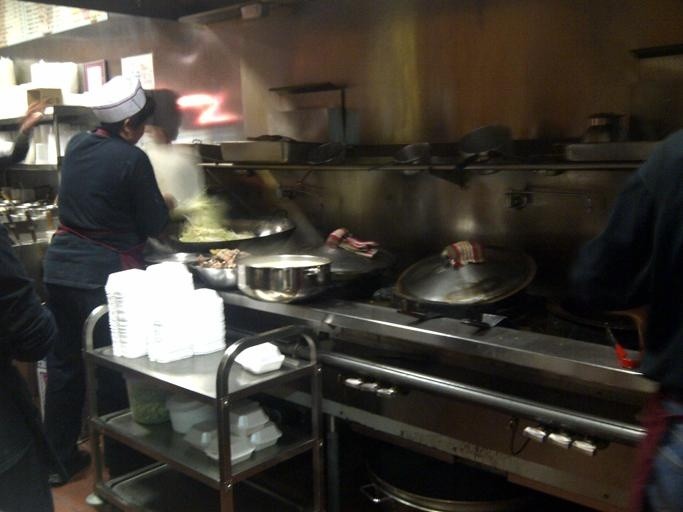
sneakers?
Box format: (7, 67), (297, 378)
(48, 450), (91, 487)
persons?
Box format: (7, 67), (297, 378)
(129, 87), (208, 212)
(0, 222), (69, 511)
(587, 128), (682, 511)
(34, 73), (192, 490)
(0, 99), (45, 173)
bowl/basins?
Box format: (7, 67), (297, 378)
(0, 198), (61, 220)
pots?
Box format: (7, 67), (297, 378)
(393, 239), (539, 325)
(448, 122), (512, 172)
(184, 256), (238, 288)
(145, 251), (209, 271)
(366, 140), (433, 172)
(295, 140), (346, 186)
(145, 211), (298, 255)
(308, 240), (398, 304)
(236, 255), (338, 303)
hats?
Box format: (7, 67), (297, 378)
(90, 75), (147, 124)
(628, 42), (683, 74)
